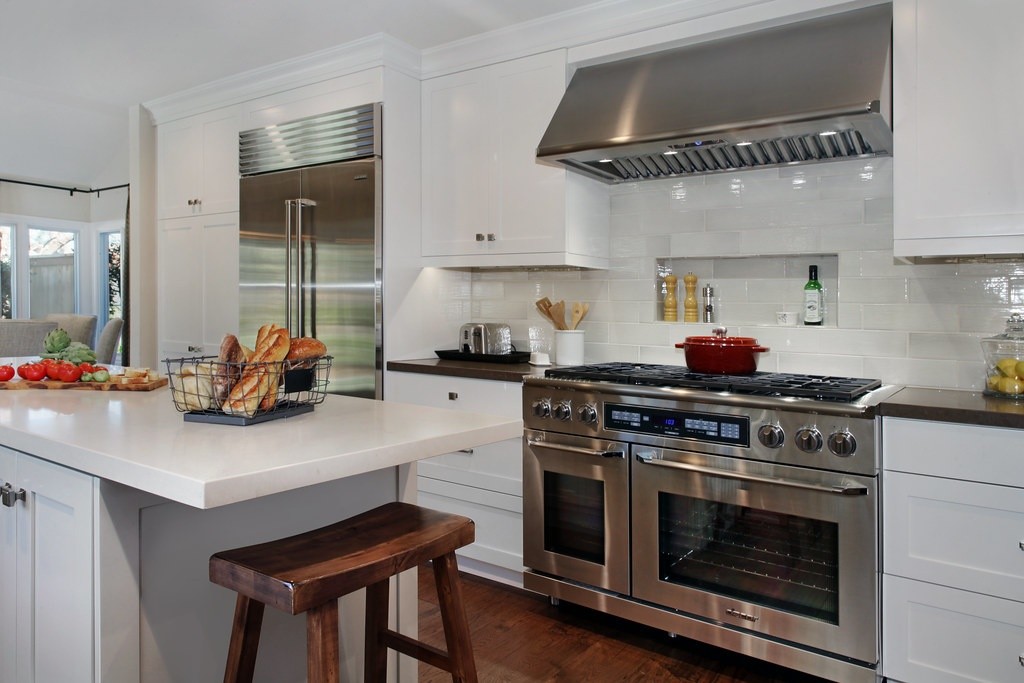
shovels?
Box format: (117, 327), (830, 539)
(536, 297), (559, 329)
(571, 303), (588, 329)
(549, 300), (568, 329)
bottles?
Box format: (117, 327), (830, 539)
(702, 283), (715, 323)
(980, 312), (1024, 401)
(803, 264), (824, 325)
(684, 271), (698, 323)
(664, 272), (677, 322)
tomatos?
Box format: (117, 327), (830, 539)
(0, 357), (112, 382)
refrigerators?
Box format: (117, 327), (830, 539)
(238, 103), (383, 400)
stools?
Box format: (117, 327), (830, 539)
(209, 502), (479, 683)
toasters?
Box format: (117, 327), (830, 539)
(458, 321), (511, 355)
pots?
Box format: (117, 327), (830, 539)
(675, 326), (770, 376)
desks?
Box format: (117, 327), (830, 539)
(0, 357), (523, 683)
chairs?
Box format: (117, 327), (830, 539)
(0, 314), (123, 365)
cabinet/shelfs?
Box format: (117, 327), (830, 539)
(880, 388), (1024, 683)
(158, 114), (239, 375)
(893, 0), (1024, 256)
(0, 447), (93, 683)
(420, 48), (610, 270)
(386, 354), (573, 597)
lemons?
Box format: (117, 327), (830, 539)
(987, 356), (1024, 394)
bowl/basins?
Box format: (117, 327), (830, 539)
(776, 312), (799, 325)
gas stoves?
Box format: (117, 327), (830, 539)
(520, 360), (907, 476)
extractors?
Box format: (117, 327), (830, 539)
(535, 3), (894, 184)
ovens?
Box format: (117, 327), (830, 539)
(521, 428), (884, 682)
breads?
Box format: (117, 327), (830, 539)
(110, 367), (159, 385)
(172, 322), (326, 418)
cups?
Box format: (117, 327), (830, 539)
(554, 329), (586, 366)
